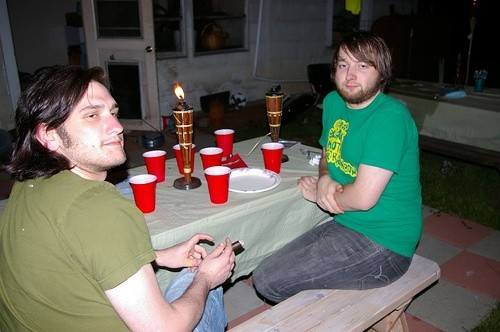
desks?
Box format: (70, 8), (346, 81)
(384, 79), (500, 153)
(0, 135), (335, 294)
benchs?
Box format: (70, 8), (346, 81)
(224, 252), (441, 332)
(418, 134), (500, 170)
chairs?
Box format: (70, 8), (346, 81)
(301, 63), (336, 125)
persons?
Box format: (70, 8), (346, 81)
(252, 31), (423, 303)
(0, 65), (235, 332)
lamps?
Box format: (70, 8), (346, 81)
(266, 85), (289, 163)
(172, 84), (203, 191)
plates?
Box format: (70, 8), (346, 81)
(228, 167), (282, 194)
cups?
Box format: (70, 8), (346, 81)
(199, 147), (224, 176)
(172, 144), (196, 175)
(214, 129), (234, 158)
(203, 166), (231, 204)
(261, 143), (284, 174)
(143, 150), (166, 183)
(129, 174), (158, 214)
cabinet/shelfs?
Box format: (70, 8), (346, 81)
(153, 0), (250, 60)
